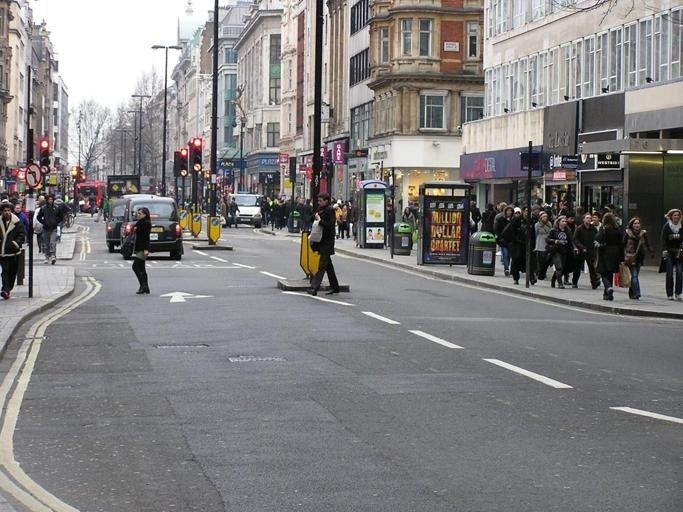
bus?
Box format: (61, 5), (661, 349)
(75, 180), (105, 213)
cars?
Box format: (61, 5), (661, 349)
(120, 194), (157, 199)
(103, 198), (129, 252)
(116, 196), (184, 261)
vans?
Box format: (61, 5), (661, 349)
(225, 194), (262, 228)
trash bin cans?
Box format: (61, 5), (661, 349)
(288, 211), (301, 233)
(390, 222), (411, 255)
(468, 231), (496, 276)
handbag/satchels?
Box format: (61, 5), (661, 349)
(620, 263), (633, 289)
(120, 233), (134, 259)
(624, 253), (637, 266)
(546, 243), (559, 251)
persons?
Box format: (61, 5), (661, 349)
(0, 191), (110, 300)
(658, 209), (683, 300)
(261, 195), (358, 240)
(367, 229), (375, 240)
(306, 192), (340, 296)
(469, 198), (655, 300)
(374, 229), (383, 240)
(387, 199), (420, 247)
(184, 197), (241, 228)
(131, 208), (151, 294)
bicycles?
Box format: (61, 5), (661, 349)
(61, 213), (74, 228)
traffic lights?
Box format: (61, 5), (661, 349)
(77, 167), (83, 181)
(190, 137), (202, 172)
(180, 149), (188, 177)
(39, 139), (50, 166)
(324, 161), (333, 180)
(72, 166), (77, 179)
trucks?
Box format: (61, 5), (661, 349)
(139, 177), (155, 194)
(102, 175), (140, 205)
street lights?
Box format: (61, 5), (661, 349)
(107, 110), (144, 176)
(131, 95), (150, 177)
(151, 46), (182, 197)
(231, 114), (242, 193)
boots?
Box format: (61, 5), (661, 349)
(136, 272), (150, 295)
